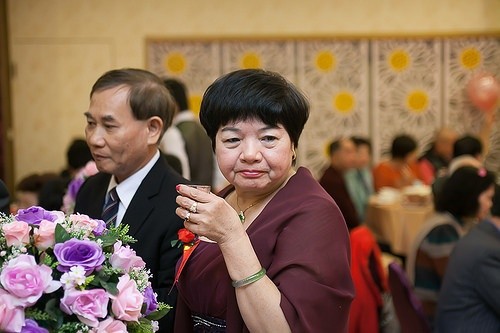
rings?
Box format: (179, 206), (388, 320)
(184, 212), (191, 221)
(188, 201), (198, 213)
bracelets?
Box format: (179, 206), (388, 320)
(231, 267), (266, 288)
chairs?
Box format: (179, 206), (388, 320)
(386, 262), (433, 333)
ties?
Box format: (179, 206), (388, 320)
(101, 186), (121, 230)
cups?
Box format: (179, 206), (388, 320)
(182, 185), (212, 211)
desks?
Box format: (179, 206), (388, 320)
(365, 191), (434, 258)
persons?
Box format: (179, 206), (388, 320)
(158, 68), (356, 333)
(319, 132), (500, 333)
(0, 68), (233, 333)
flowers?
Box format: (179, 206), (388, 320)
(0, 204), (173, 333)
(171, 228), (200, 251)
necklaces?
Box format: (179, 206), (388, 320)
(235, 190), (275, 225)
